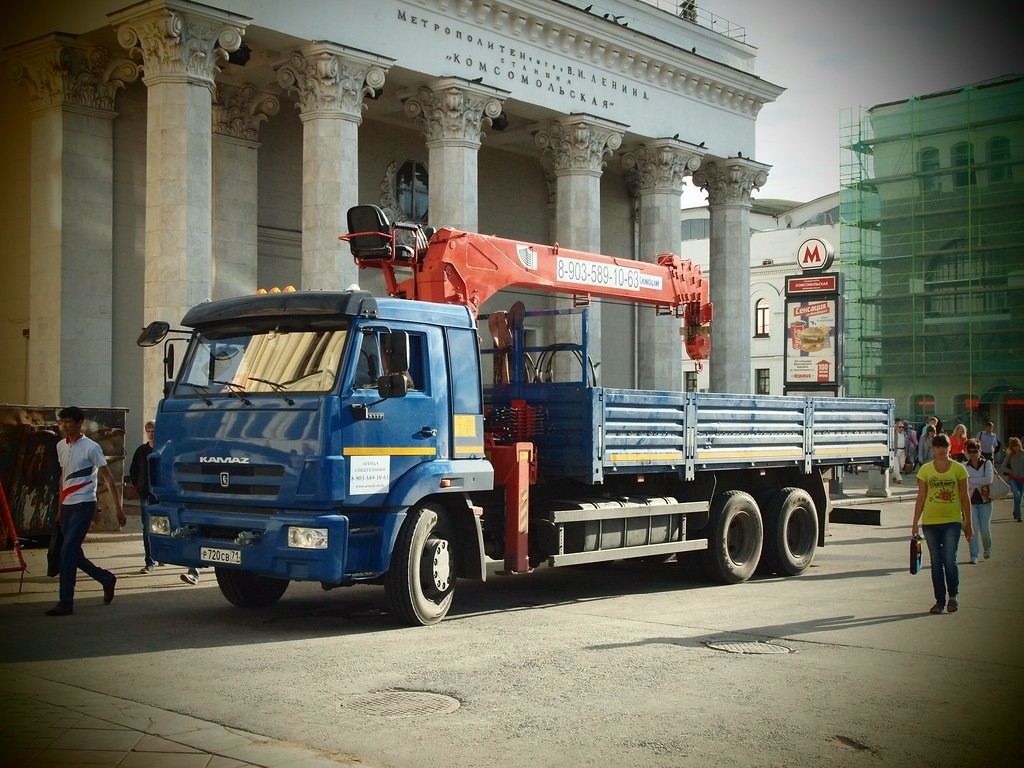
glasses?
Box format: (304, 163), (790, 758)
(968, 449), (980, 454)
(899, 426), (904, 428)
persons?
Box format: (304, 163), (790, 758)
(912, 433), (973, 613)
(46, 405), (126, 616)
(180, 568), (199, 586)
(369, 340), (415, 390)
(891, 416), (1024, 523)
(962, 437), (994, 564)
(128, 419), (165, 575)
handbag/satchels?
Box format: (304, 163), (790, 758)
(984, 459), (1011, 501)
(47, 522), (64, 577)
(910, 536), (922, 574)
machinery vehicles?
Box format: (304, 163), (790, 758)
(138, 204), (894, 624)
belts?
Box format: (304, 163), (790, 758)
(897, 447), (905, 449)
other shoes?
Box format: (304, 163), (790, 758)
(969, 558), (977, 564)
(140, 565), (155, 574)
(984, 547), (992, 559)
(103, 574), (116, 605)
(1017, 517), (1022, 522)
(45, 606), (73, 616)
(180, 573), (198, 585)
(893, 477), (903, 484)
(1014, 512), (1017, 519)
(947, 595), (959, 612)
(930, 605), (944, 614)
